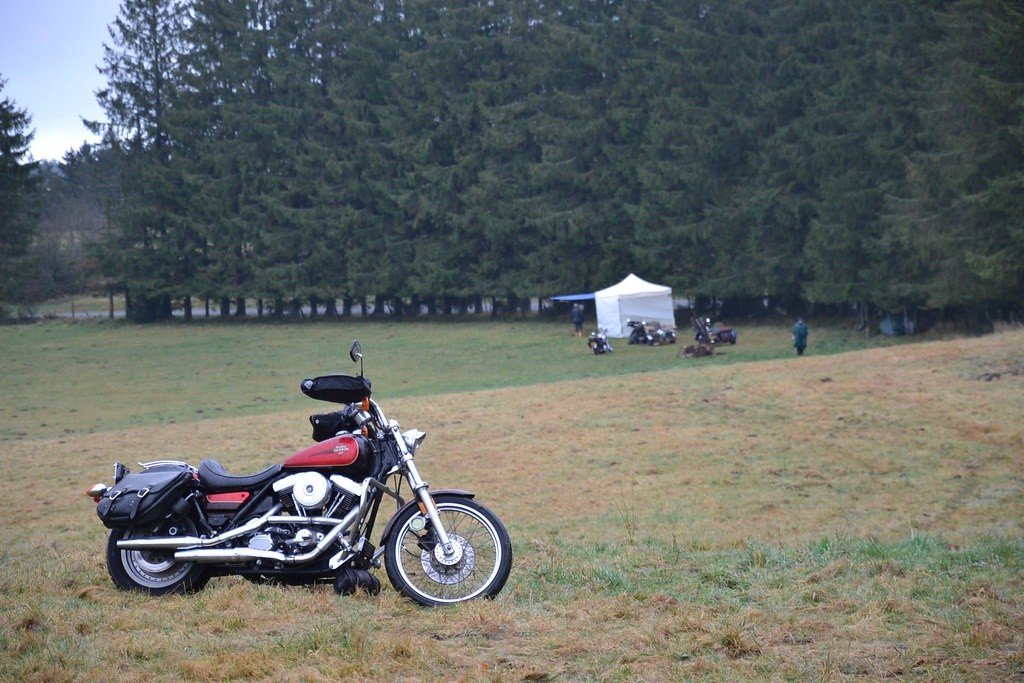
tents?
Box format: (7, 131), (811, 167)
(549, 272), (676, 338)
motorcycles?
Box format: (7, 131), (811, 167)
(87, 339), (515, 606)
(625, 319), (676, 346)
(713, 322), (737, 345)
(587, 327), (613, 356)
(692, 317), (720, 345)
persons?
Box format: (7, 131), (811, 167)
(792, 317), (809, 356)
(571, 303), (585, 338)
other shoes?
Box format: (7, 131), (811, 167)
(579, 333), (581, 336)
(575, 332), (578, 336)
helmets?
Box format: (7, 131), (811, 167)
(574, 303), (578, 308)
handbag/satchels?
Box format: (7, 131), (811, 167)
(96, 471), (193, 527)
